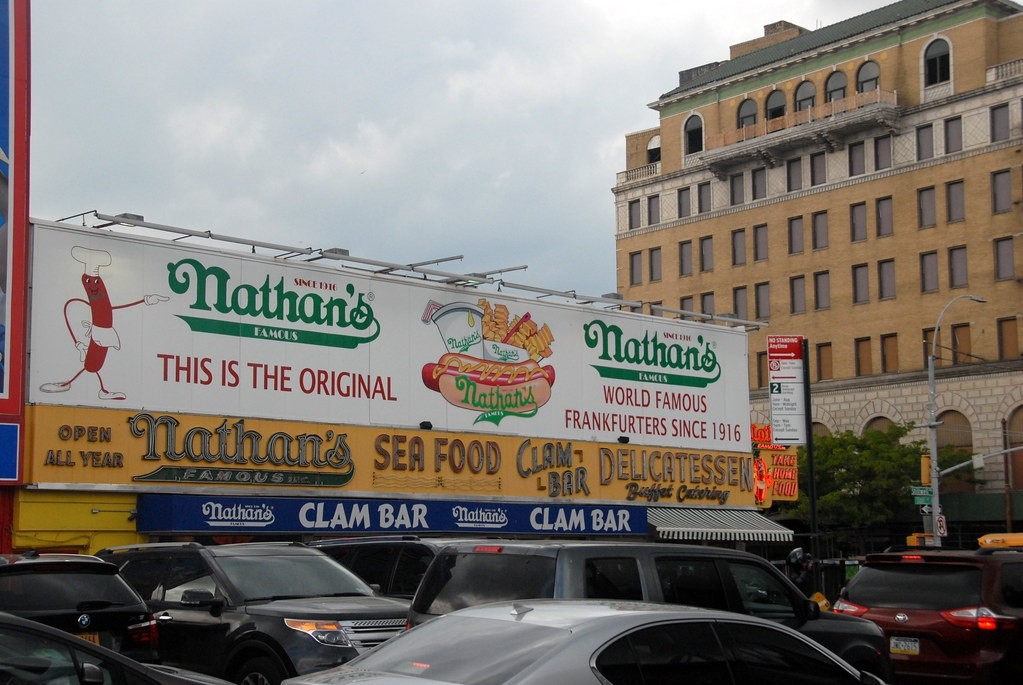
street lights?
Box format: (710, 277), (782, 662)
(924, 294), (989, 548)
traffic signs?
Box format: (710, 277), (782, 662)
(765, 334), (809, 447)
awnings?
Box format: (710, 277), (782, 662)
(646, 508), (796, 545)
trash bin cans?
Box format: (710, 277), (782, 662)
(820, 558), (860, 602)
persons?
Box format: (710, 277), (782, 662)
(788, 544), (811, 583)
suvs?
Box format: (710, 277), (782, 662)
(831, 546), (1022, 685)
(287, 543), (891, 685)
(2, 537), (497, 685)
